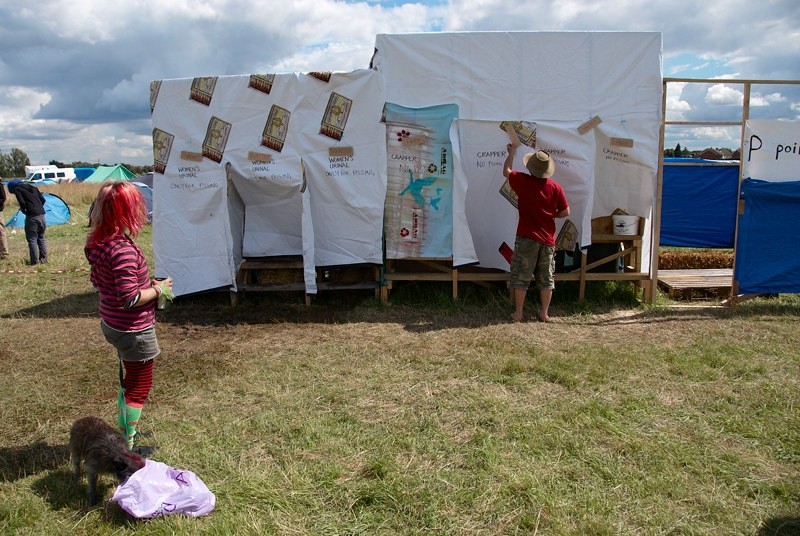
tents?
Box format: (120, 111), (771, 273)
(35, 179), (55, 184)
(4, 193), (71, 227)
(82, 164), (138, 183)
(132, 172), (153, 189)
(132, 182), (153, 224)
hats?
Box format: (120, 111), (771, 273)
(7, 178), (22, 194)
(523, 149), (555, 178)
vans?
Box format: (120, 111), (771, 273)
(21, 167), (77, 183)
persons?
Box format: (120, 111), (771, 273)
(0, 180), (9, 258)
(503, 142), (570, 322)
(7, 179), (46, 266)
(83, 181), (173, 458)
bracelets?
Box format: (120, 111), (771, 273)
(153, 285), (162, 297)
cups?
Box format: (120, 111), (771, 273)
(151, 278), (167, 311)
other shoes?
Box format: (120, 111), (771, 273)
(27, 262), (36, 265)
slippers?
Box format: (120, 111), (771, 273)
(507, 313), (520, 322)
(537, 309), (547, 323)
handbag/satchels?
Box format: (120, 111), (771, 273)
(110, 458), (216, 518)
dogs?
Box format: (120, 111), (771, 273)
(69, 417), (144, 506)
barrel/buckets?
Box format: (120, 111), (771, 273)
(612, 214), (641, 236)
